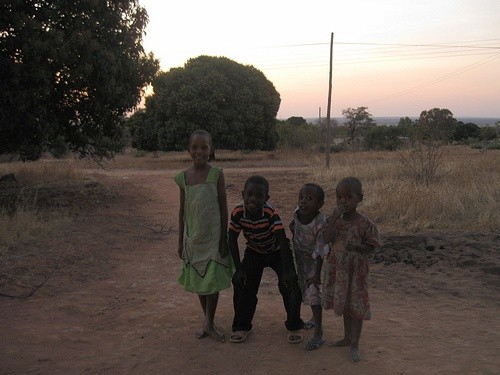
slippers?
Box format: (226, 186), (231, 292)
(287, 332), (303, 344)
(304, 320), (316, 330)
(230, 329), (251, 343)
(303, 339), (327, 350)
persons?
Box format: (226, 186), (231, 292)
(322, 176), (382, 361)
(289, 183), (331, 351)
(228, 176), (303, 344)
(172, 130), (226, 344)
